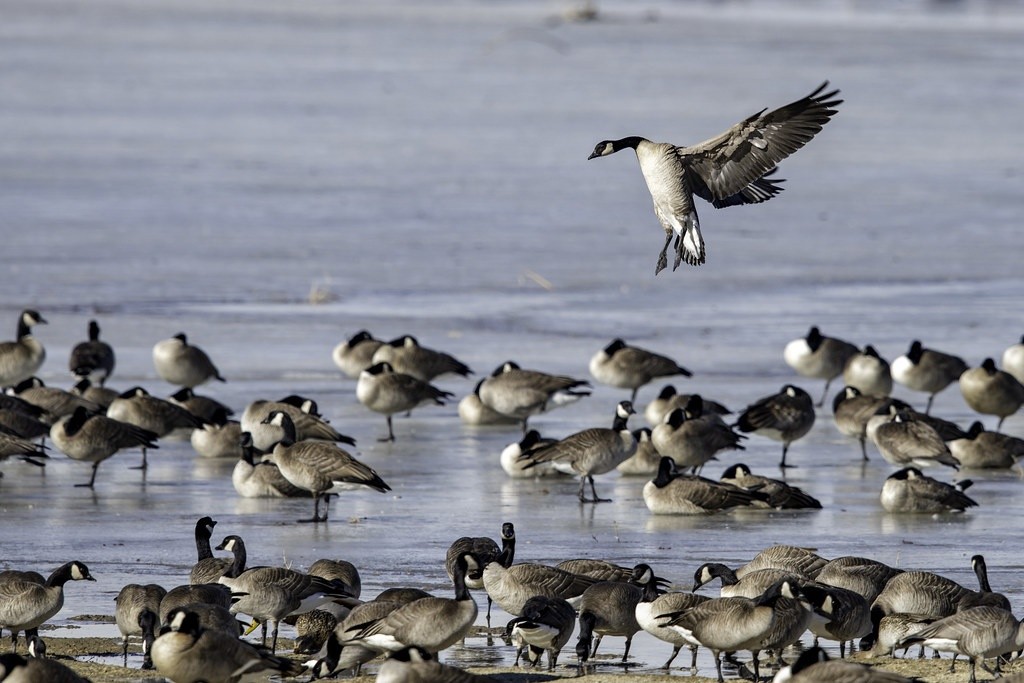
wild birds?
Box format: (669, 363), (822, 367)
(459, 361), (596, 440)
(616, 325), (1024, 516)
(231, 431), (339, 498)
(0, 515), (1024, 683)
(106, 387), (205, 470)
(590, 338), (694, 404)
(587, 79), (844, 276)
(239, 394), (357, 454)
(513, 401), (637, 504)
(169, 387), (236, 426)
(68, 321), (115, 386)
(152, 333), (226, 386)
(189, 417), (242, 457)
(332, 329), (384, 379)
(0, 309), (106, 479)
(372, 334), (476, 419)
(499, 430), (570, 479)
(47, 405), (160, 487)
(260, 411), (392, 523)
(356, 363), (455, 442)
